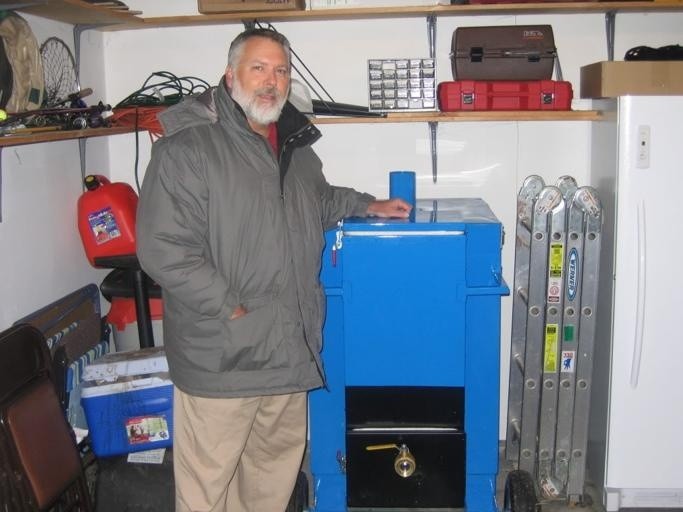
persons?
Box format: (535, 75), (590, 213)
(135, 30), (413, 512)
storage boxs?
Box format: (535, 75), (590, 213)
(78, 345), (172, 458)
(580, 61), (683, 98)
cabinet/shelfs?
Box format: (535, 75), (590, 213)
(0, 0), (683, 192)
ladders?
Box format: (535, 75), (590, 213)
(505, 175), (604, 503)
